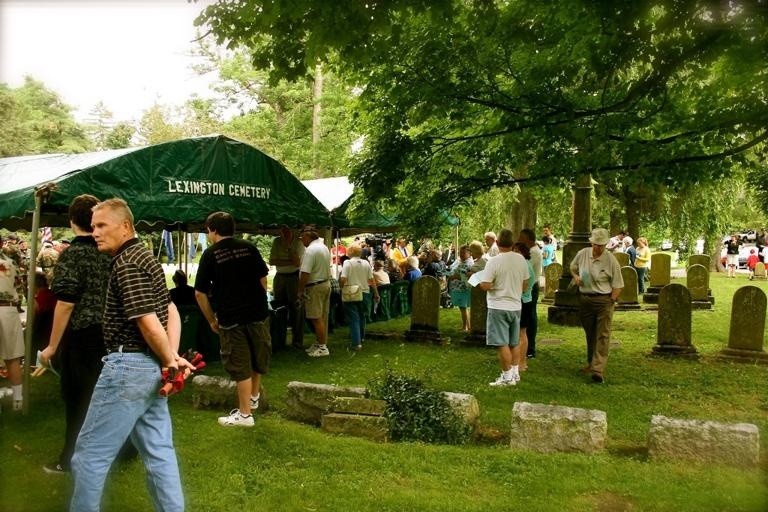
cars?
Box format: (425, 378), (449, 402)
(660, 226), (768, 270)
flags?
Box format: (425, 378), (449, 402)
(198, 232), (208, 255)
(184, 230), (196, 262)
(161, 229), (175, 267)
(39, 226), (52, 246)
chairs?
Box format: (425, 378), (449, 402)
(171, 269), (411, 369)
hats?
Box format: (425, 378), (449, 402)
(469, 231), (498, 250)
(589, 228), (610, 245)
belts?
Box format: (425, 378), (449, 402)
(303, 279), (329, 287)
(106, 345), (152, 357)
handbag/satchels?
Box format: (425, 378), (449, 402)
(342, 285), (364, 302)
(567, 274), (581, 297)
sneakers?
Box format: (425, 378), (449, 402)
(250, 392), (259, 409)
(12, 397), (23, 410)
(306, 341), (330, 357)
(489, 374), (520, 386)
(218, 408), (257, 429)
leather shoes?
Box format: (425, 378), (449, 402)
(349, 342), (362, 350)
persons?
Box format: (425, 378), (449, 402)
(29, 196), (137, 475)
(69, 194), (197, 512)
(480, 228), (543, 389)
(723, 225), (768, 280)
(268, 224), (498, 372)
(569, 228), (624, 385)
(609, 232), (651, 293)
(0, 233), (72, 410)
(167, 270), (195, 316)
(192, 212), (269, 427)
(540, 226), (557, 266)
